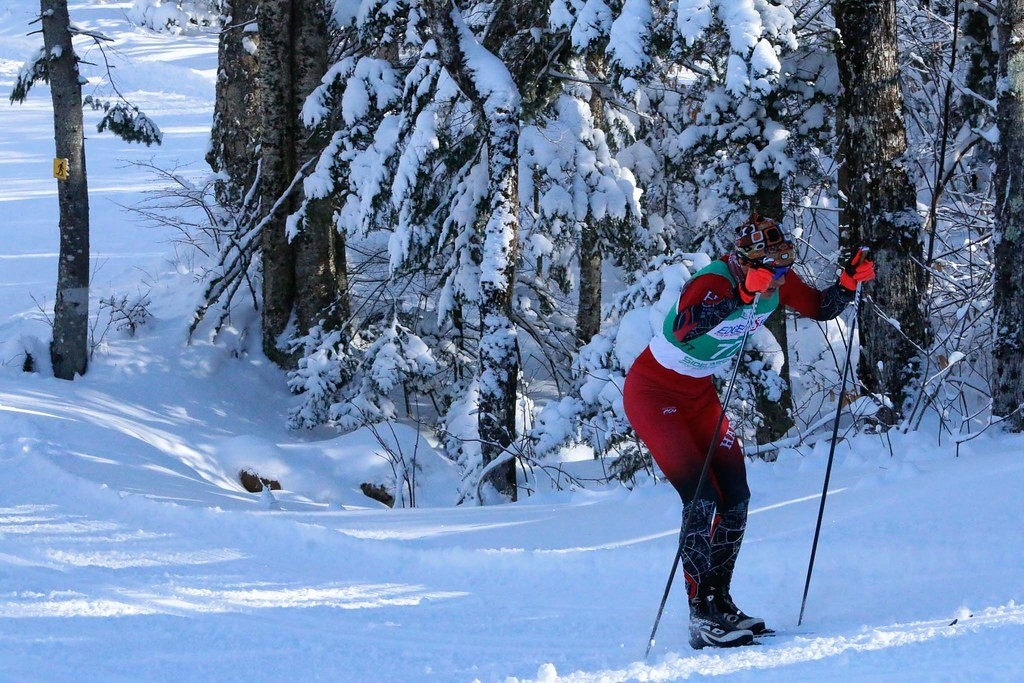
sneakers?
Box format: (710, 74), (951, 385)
(688, 603), (753, 650)
(718, 602), (765, 635)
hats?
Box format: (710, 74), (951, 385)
(734, 212), (795, 267)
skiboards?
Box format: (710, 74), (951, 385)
(752, 612), (974, 647)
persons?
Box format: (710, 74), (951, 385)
(623, 212), (876, 649)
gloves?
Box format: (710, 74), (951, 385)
(736, 267), (774, 304)
(838, 249), (875, 298)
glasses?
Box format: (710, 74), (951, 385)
(772, 264), (793, 281)
